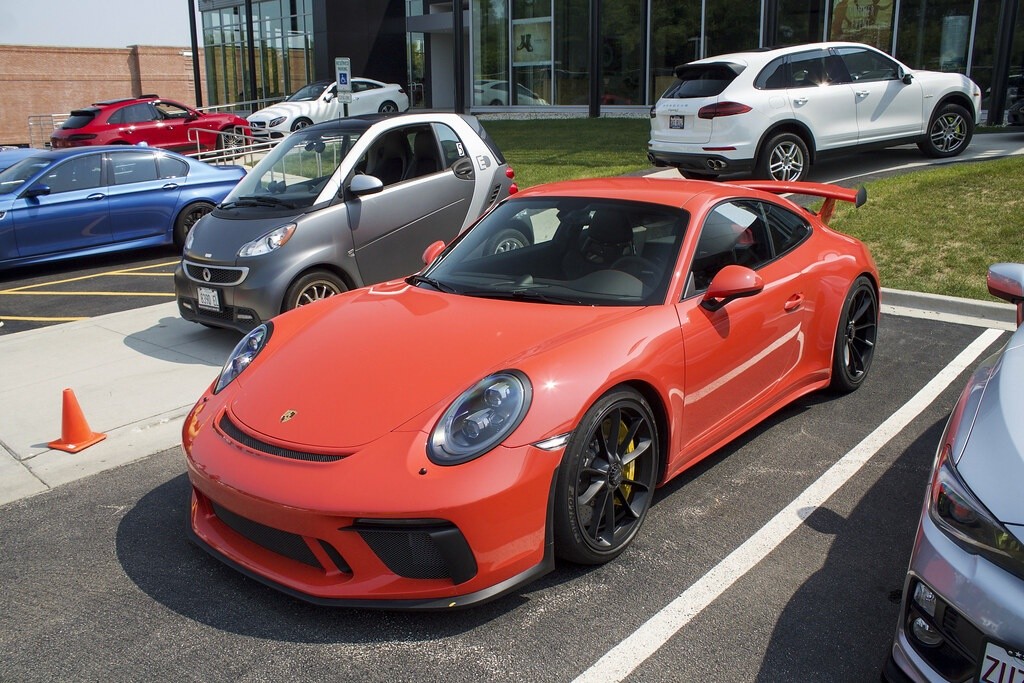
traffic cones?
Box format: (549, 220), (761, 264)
(47, 388), (107, 454)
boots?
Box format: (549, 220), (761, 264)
(517, 35), (525, 50)
(525, 34), (533, 52)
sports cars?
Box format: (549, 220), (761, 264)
(879, 261), (1024, 683)
(181, 176), (880, 612)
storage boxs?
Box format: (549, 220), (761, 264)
(655, 76), (676, 103)
(543, 77), (579, 105)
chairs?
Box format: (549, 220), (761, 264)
(565, 210), (636, 280)
(70, 157), (100, 191)
(669, 212), (738, 290)
(402, 131), (441, 181)
(48, 165), (71, 194)
(803, 60), (828, 86)
(368, 131), (412, 185)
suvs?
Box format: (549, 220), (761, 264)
(647, 40), (983, 190)
(49, 93), (254, 160)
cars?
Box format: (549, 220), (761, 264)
(0, 147), (62, 193)
(573, 92), (641, 106)
(245, 77), (411, 144)
(926, 56), (1024, 125)
(472, 78), (550, 107)
(174, 110), (535, 344)
(0, 144), (250, 270)
